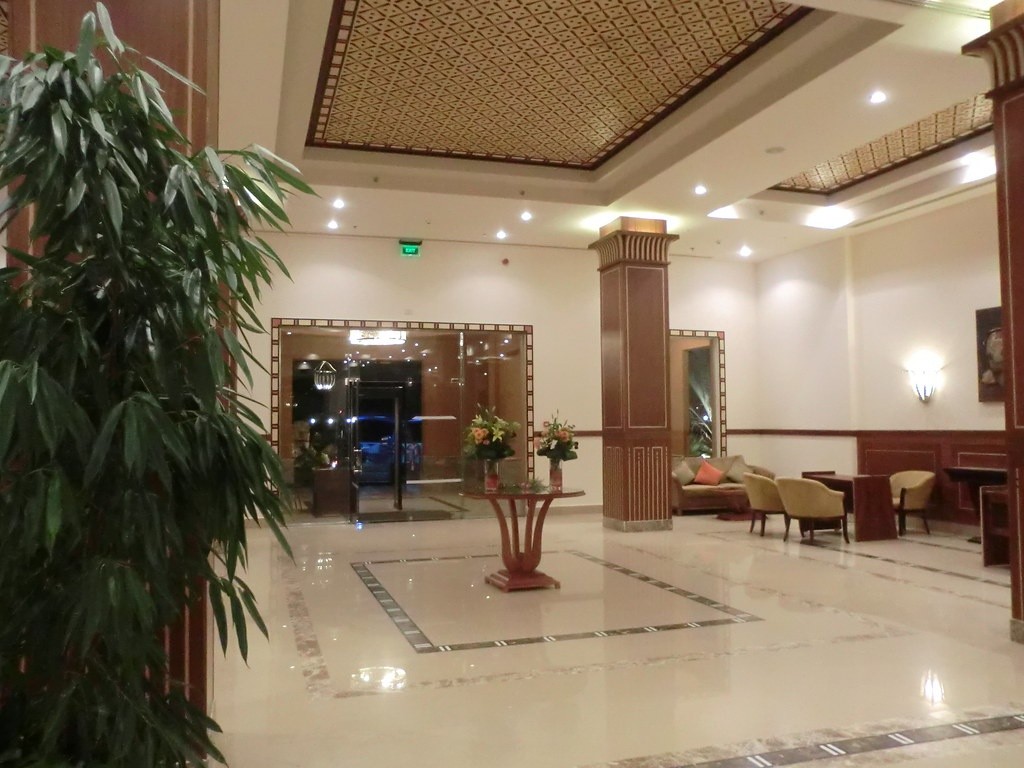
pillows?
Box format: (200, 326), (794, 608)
(694, 460), (724, 485)
(672, 459), (696, 485)
(726, 458), (754, 484)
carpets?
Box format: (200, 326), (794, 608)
(357, 509), (452, 523)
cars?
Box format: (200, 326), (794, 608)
(344, 414), (423, 487)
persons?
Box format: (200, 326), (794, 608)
(293, 420), (329, 485)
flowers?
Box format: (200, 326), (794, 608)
(461, 400), (522, 473)
(536, 408), (579, 467)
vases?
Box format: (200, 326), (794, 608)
(484, 460), (499, 490)
(549, 459), (563, 491)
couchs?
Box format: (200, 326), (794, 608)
(670, 454), (775, 516)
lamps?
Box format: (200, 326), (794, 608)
(312, 360), (336, 391)
(905, 350), (944, 402)
(296, 361), (311, 369)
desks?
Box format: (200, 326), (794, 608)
(459, 482), (587, 592)
(311, 465), (362, 517)
(801, 470), (897, 543)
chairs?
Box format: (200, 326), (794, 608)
(774, 476), (850, 545)
(743, 472), (806, 538)
(890, 470), (935, 538)
